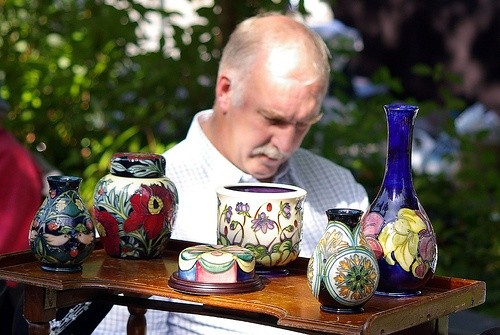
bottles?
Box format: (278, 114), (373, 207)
(360, 103), (438, 299)
(307, 206), (380, 314)
(92, 152), (179, 261)
(28, 175), (95, 273)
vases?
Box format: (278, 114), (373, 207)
(91, 151), (181, 260)
(29, 176), (97, 273)
(308, 103), (433, 317)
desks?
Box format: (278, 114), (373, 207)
(0, 240), (487, 335)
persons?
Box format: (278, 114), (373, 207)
(88, 11), (371, 335)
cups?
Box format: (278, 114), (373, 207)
(214, 181), (307, 278)
